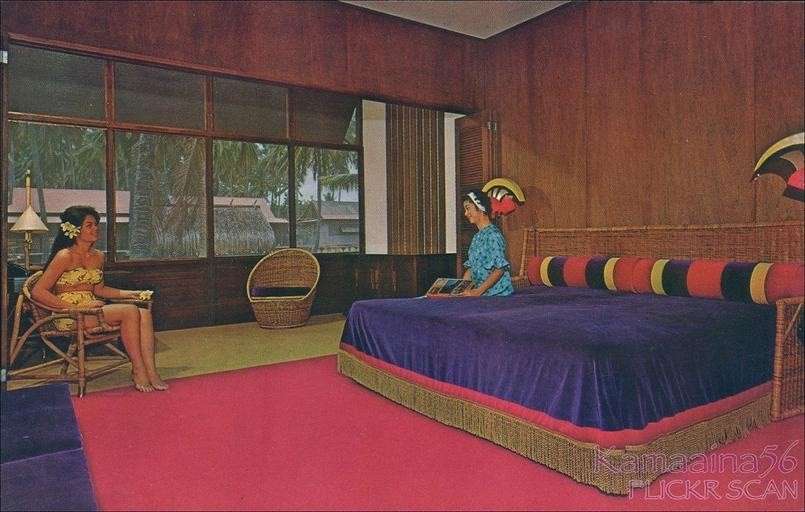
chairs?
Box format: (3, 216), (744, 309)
(246, 246), (321, 329)
(9, 270), (154, 398)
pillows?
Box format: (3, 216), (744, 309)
(527, 255), (657, 293)
(632, 252), (805, 308)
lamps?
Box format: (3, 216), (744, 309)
(10, 169), (48, 360)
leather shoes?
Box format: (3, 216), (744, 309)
(131, 368), (168, 393)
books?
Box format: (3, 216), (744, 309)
(412, 277), (475, 298)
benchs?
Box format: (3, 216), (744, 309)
(0, 382), (97, 512)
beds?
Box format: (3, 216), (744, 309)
(336, 220), (805, 496)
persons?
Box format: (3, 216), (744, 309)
(30, 205), (168, 393)
(461, 189), (516, 297)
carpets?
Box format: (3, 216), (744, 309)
(69, 353), (805, 512)
